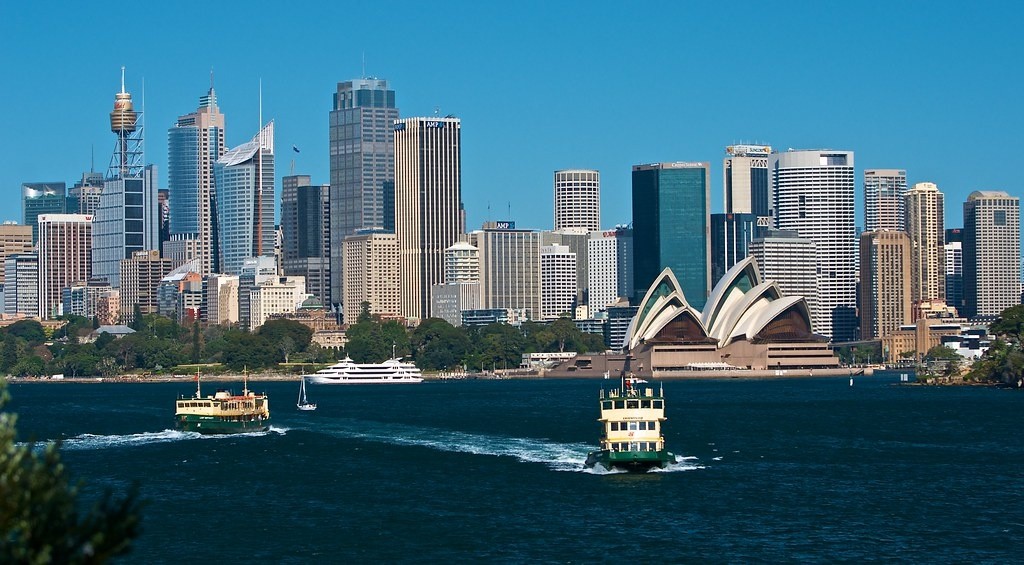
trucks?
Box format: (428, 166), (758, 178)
(51, 374), (64, 379)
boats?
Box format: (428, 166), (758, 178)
(299, 341), (425, 384)
(174, 364), (271, 438)
(297, 367), (317, 412)
(584, 371), (678, 472)
